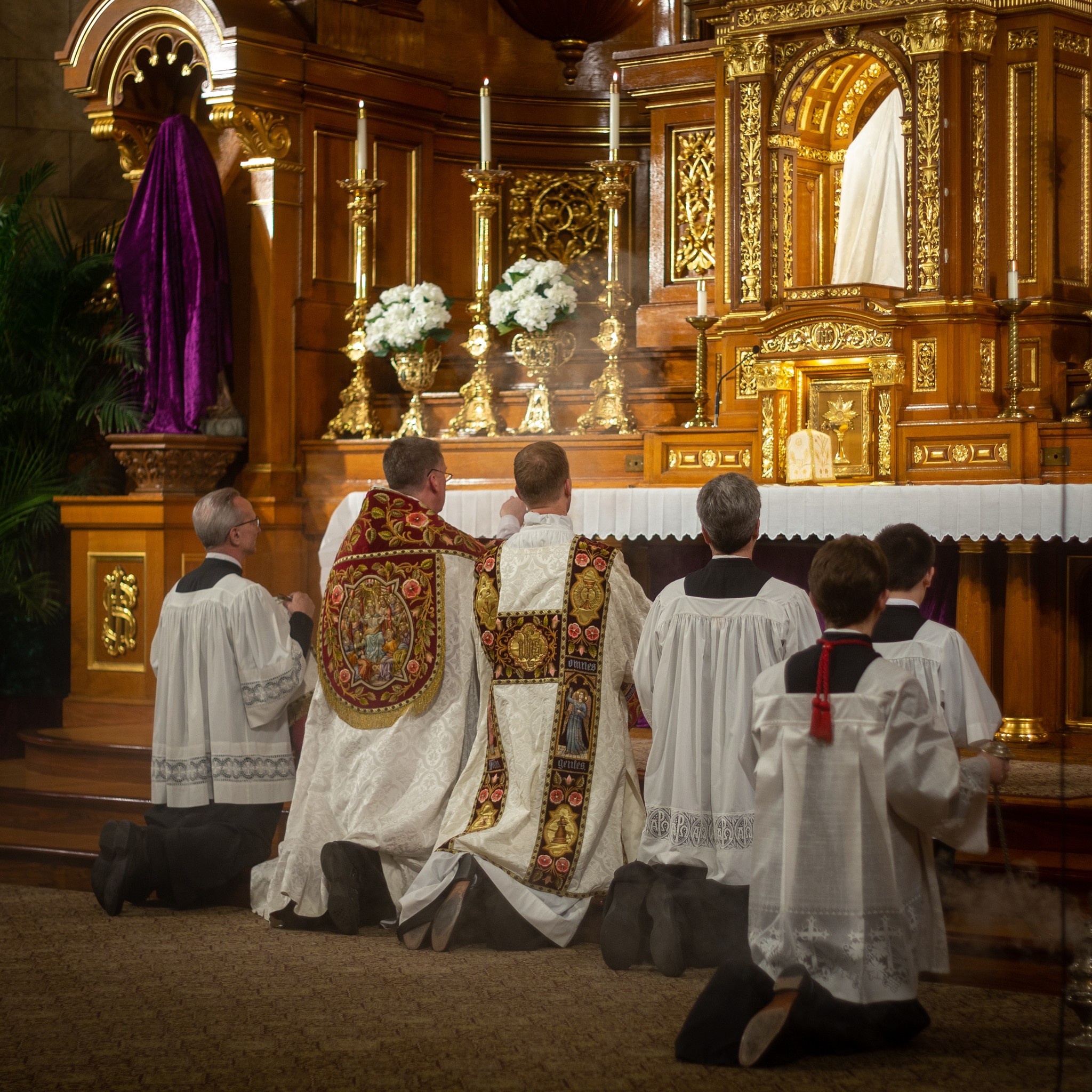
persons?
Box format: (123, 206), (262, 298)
(250, 434), (528, 935)
(599, 471), (824, 978)
(90, 487), (315, 917)
(674, 531), (1010, 1070)
(395, 441), (653, 953)
(871, 524), (1003, 858)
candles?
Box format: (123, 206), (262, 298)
(610, 70), (620, 151)
(696, 278), (706, 316)
(1007, 260), (1019, 299)
(356, 100), (368, 170)
(480, 77), (491, 162)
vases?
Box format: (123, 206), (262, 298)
(388, 349), (443, 437)
(510, 329), (577, 434)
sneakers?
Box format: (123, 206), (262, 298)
(402, 898), (438, 950)
(270, 902), (328, 929)
(600, 880), (649, 971)
(320, 840), (363, 935)
(432, 852), (480, 951)
(645, 882), (687, 978)
(739, 964), (815, 1066)
(90, 821), (150, 916)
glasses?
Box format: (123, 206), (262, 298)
(428, 468), (453, 481)
(227, 516), (260, 536)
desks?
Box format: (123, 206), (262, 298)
(316, 480), (1092, 601)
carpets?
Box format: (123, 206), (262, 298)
(628, 737), (1092, 798)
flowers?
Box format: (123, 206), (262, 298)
(488, 257), (579, 335)
(361, 280), (452, 358)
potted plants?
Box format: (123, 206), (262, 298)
(0, 158), (148, 764)
